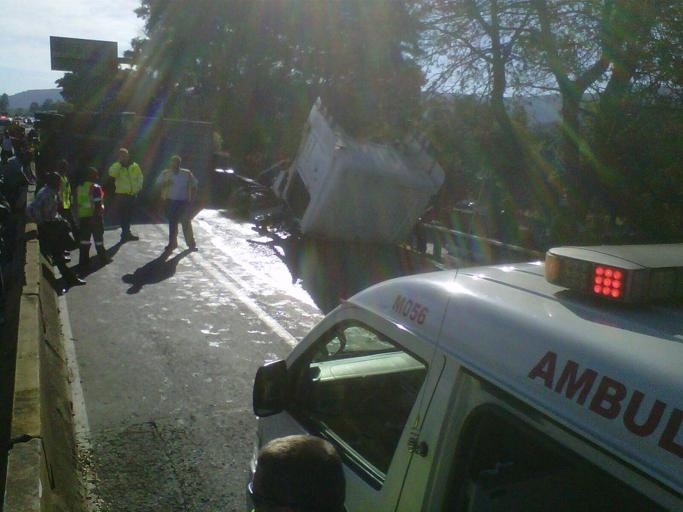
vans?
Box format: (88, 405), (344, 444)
(0, 116), (14, 138)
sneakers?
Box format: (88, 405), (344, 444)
(68, 277), (87, 285)
(164, 242), (178, 250)
(189, 245), (199, 252)
(120, 230), (139, 242)
(52, 244), (72, 266)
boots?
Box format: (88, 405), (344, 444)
(79, 241), (97, 273)
(95, 242), (114, 264)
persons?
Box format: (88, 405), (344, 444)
(248, 435), (348, 512)
(0, 119), (85, 286)
(104, 148), (146, 241)
(154, 154), (199, 251)
(73, 168), (114, 273)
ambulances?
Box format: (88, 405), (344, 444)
(248, 240), (683, 512)
